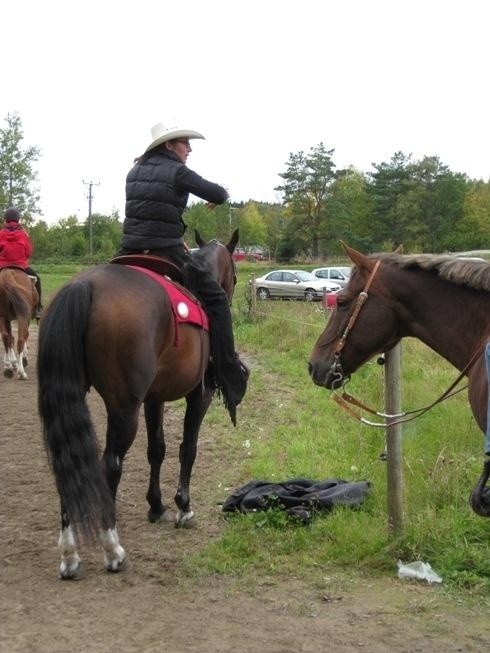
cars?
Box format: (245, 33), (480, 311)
(232, 247), (263, 262)
(311, 266), (353, 289)
(250, 269), (342, 302)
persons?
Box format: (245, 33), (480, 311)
(0, 207), (44, 319)
(111, 118), (248, 408)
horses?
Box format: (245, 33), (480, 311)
(305, 238), (489, 441)
(34, 225), (242, 583)
(0, 264), (41, 380)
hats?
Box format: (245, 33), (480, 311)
(3, 207), (21, 224)
(134, 119), (206, 159)
